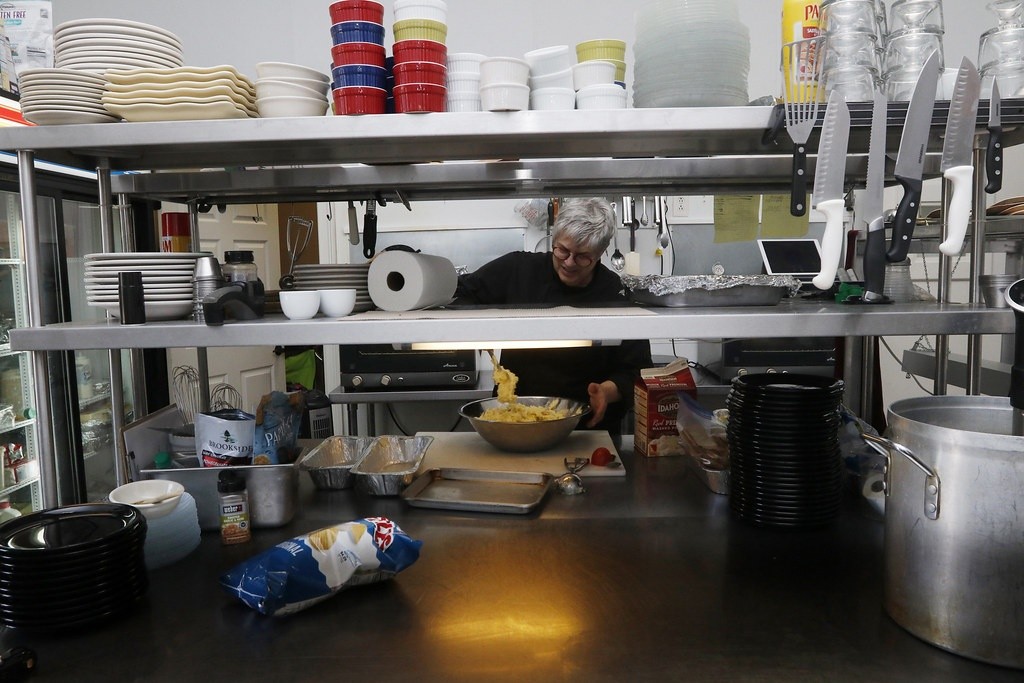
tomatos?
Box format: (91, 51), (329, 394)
(591, 447), (611, 465)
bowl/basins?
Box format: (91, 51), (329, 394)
(190, 257), (226, 321)
(109, 479), (185, 520)
(278, 290), (321, 320)
(458, 396), (592, 453)
(318, 289), (357, 318)
(979, 274), (1020, 308)
(253, 62), (331, 118)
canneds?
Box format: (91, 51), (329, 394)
(218, 470), (250, 544)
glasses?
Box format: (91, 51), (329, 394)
(552, 241), (600, 268)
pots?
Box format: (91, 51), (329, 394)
(861, 396), (1024, 670)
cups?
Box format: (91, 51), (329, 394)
(813, 0), (1024, 103)
(327, 0), (628, 115)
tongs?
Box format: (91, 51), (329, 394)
(640, 196), (649, 226)
(659, 196), (670, 248)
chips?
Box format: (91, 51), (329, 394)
(309, 523), (364, 550)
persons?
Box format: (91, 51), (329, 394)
(454, 195), (654, 433)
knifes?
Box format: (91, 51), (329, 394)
(984, 75), (1004, 194)
(811, 90), (851, 290)
(938, 56), (981, 257)
(860, 90), (888, 302)
(886, 48), (939, 262)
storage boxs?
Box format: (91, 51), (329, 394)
(0, 0), (55, 128)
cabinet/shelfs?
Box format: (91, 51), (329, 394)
(1, 106), (1024, 513)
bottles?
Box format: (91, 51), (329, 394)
(0, 501), (22, 524)
(162, 212), (191, 254)
(75, 351), (94, 398)
(215, 470), (251, 544)
(15, 407), (34, 421)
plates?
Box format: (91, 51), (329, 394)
(292, 264), (373, 311)
(725, 373), (846, 530)
(83, 251), (214, 321)
(0, 502), (147, 631)
(18, 18), (260, 125)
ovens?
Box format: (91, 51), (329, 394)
(720, 336), (843, 382)
(338, 339), (481, 392)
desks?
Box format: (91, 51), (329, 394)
(328, 366), (735, 436)
(0, 434), (1024, 683)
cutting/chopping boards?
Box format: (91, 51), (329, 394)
(411, 430), (626, 477)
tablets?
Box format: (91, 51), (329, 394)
(758, 238), (822, 276)
